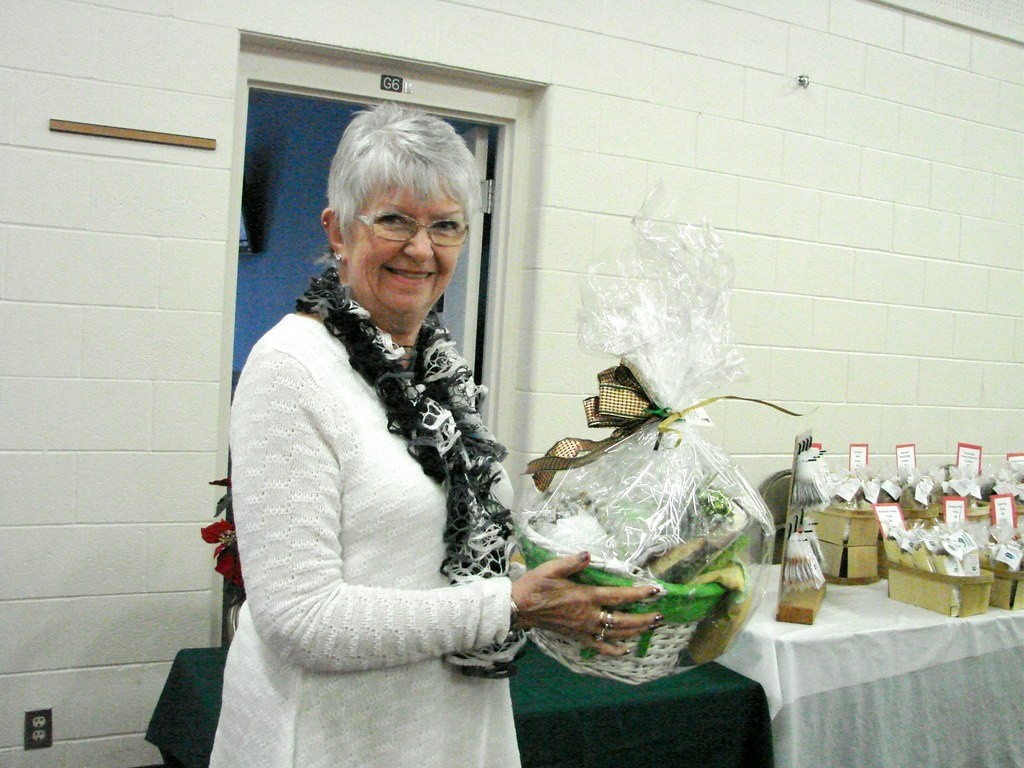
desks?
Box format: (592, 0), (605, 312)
(714, 565), (1024, 768)
(142, 637), (775, 768)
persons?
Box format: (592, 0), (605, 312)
(207, 102), (667, 768)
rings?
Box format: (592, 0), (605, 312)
(595, 628), (605, 641)
(599, 610), (605, 623)
(605, 612), (613, 629)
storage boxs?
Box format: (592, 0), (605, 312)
(808, 499), (1024, 617)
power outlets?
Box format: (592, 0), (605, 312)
(24, 707), (52, 750)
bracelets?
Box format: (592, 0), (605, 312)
(511, 598), (519, 631)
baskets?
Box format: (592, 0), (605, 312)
(508, 510), (723, 684)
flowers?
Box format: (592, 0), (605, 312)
(200, 476), (244, 599)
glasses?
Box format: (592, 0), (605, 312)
(353, 211), (471, 248)
